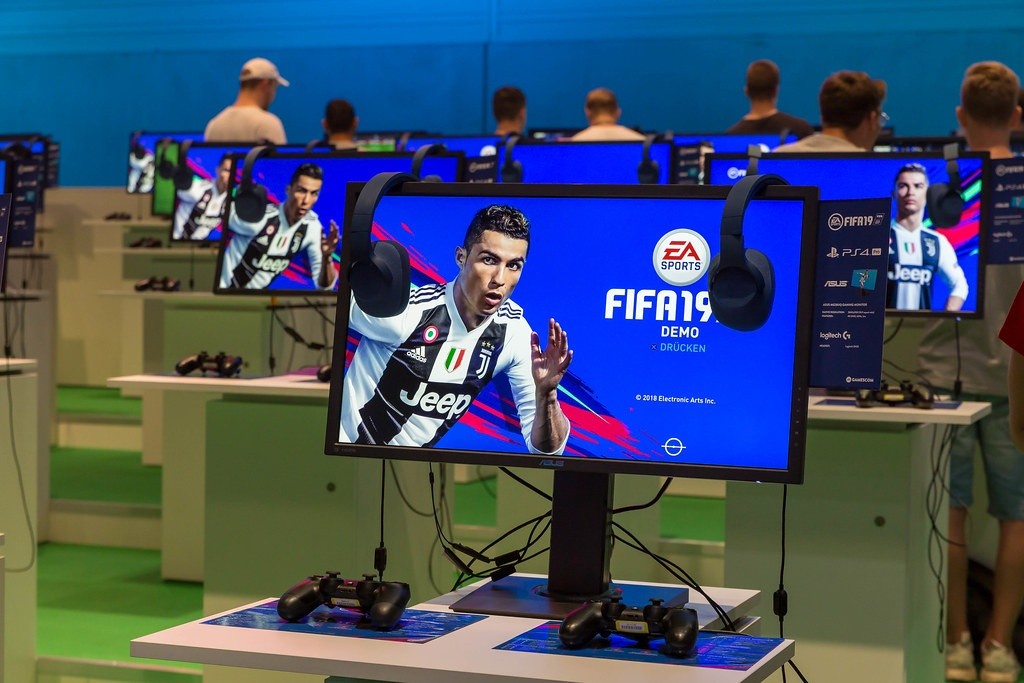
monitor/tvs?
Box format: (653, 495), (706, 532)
(126, 125), (1024, 620)
(0, 133), (60, 297)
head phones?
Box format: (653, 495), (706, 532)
(132, 128), (147, 161)
(708, 174), (793, 330)
(348, 171), (423, 317)
(159, 137), (173, 179)
(501, 134), (524, 182)
(409, 143), (448, 182)
(306, 138), (324, 155)
(174, 138), (193, 190)
(637, 135), (660, 184)
(745, 142), (761, 176)
(924, 143), (963, 228)
(235, 146), (275, 223)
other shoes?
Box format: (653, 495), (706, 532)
(945, 632), (977, 681)
(981, 639), (1020, 683)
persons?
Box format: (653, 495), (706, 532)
(217, 163), (339, 290)
(919, 62), (1024, 683)
(727, 60), (813, 137)
(773, 71), (885, 152)
(205, 57), (286, 146)
(493, 87), (527, 135)
(886, 164), (969, 310)
(997, 280), (1024, 456)
(570, 88), (646, 140)
(322, 101), (359, 150)
(339, 204), (574, 455)
(173, 153), (232, 239)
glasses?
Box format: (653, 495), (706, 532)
(868, 109), (890, 127)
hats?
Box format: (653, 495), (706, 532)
(239, 57), (290, 87)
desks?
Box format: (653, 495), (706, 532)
(1, 214), (995, 683)
